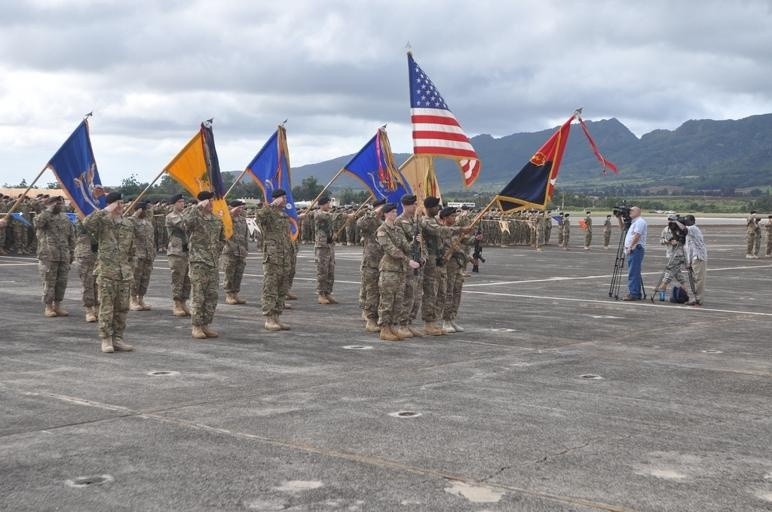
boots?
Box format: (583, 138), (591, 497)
(318, 294), (336, 304)
(138, 295), (151, 309)
(84, 306), (99, 321)
(0, 247), (30, 256)
(366, 318), (381, 331)
(424, 317), (464, 336)
(286, 292), (297, 300)
(192, 325), (205, 338)
(226, 293), (244, 304)
(53, 301), (68, 316)
(113, 337), (133, 351)
(380, 319), (423, 340)
(659, 282), (667, 290)
(101, 337), (114, 352)
(285, 303), (291, 308)
(45, 303), (56, 317)
(202, 324), (217, 337)
(173, 300), (191, 316)
(265, 313), (290, 330)
(128, 296), (142, 311)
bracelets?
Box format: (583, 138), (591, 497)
(629, 246), (632, 248)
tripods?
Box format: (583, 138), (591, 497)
(651, 241), (702, 307)
(608, 228), (647, 299)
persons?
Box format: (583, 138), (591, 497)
(127, 201), (155, 311)
(584, 211), (592, 250)
(669, 214), (708, 306)
(764, 215), (772, 257)
(745, 210), (757, 259)
(753, 216), (763, 259)
(81, 191), (138, 354)
(284, 301), (292, 309)
(180, 191), (225, 340)
(355, 193), (485, 343)
(615, 206), (648, 301)
(0, 191), (556, 258)
(164, 193), (192, 317)
(223, 200), (249, 305)
(73, 216), (100, 322)
(34, 195), (77, 318)
(562, 214), (571, 251)
(279, 236), (299, 300)
(313, 196), (356, 303)
(558, 212), (564, 246)
(254, 189), (293, 332)
(603, 215), (612, 249)
(655, 212), (688, 291)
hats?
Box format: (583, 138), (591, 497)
(198, 191), (214, 200)
(271, 189), (286, 197)
(749, 210), (772, 220)
(424, 197), (440, 207)
(318, 198), (328, 205)
(373, 198), (397, 213)
(0, 193), (64, 204)
(401, 194), (416, 205)
(457, 205), (481, 213)
(229, 201), (245, 207)
(134, 202), (147, 211)
(106, 192), (123, 202)
(169, 194), (182, 204)
(441, 207), (456, 216)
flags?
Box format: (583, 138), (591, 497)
(162, 121), (234, 239)
(47, 118), (108, 221)
(341, 129), (415, 217)
(578, 218), (587, 229)
(246, 126), (300, 241)
(406, 51), (481, 189)
(493, 113), (577, 216)
(552, 214), (563, 224)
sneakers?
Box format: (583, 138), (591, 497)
(623, 294), (641, 301)
(745, 253), (772, 259)
